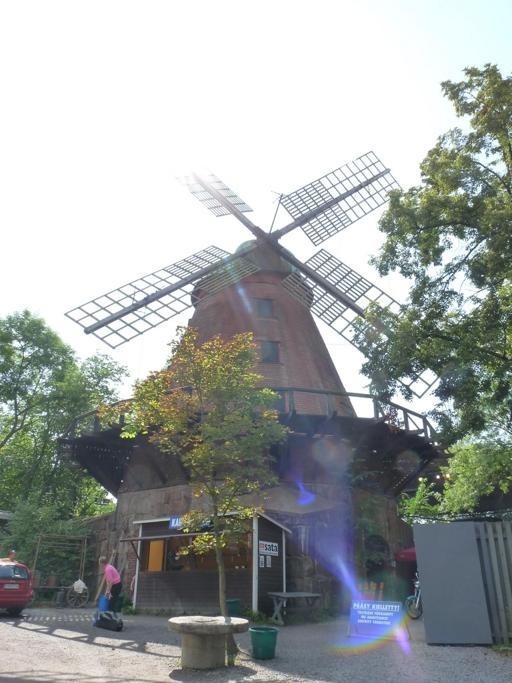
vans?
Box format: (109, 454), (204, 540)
(0, 551), (33, 617)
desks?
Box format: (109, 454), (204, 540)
(167, 615), (249, 670)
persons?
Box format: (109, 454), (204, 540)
(0, 549), (18, 562)
(99, 555), (122, 612)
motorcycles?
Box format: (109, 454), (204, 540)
(405, 569), (423, 620)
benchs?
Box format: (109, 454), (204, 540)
(268, 591), (327, 626)
